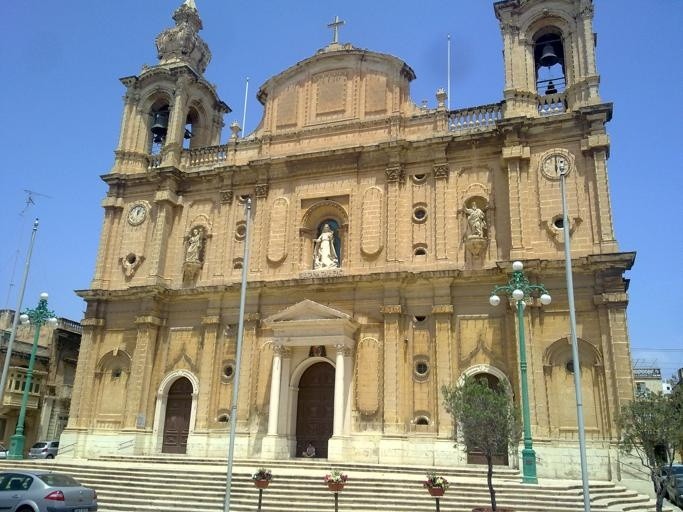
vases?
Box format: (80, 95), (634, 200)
(427, 486), (445, 496)
(255, 479), (269, 488)
(327, 482), (345, 491)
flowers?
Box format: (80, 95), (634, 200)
(324, 468), (349, 484)
(251, 466), (272, 482)
(423, 471), (450, 492)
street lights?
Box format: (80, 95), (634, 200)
(490, 261), (551, 484)
(7, 293), (58, 460)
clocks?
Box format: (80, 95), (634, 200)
(128, 204), (147, 224)
(541, 152), (571, 178)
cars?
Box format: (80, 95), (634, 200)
(0, 445), (7, 458)
(27, 441), (59, 459)
(0, 470), (97, 512)
(654, 464), (683, 509)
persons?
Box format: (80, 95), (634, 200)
(462, 201), (484, 239)
(301, 442), (315, 458)
(186, 228), (199, 263)
(312, 223), (338, 269)
